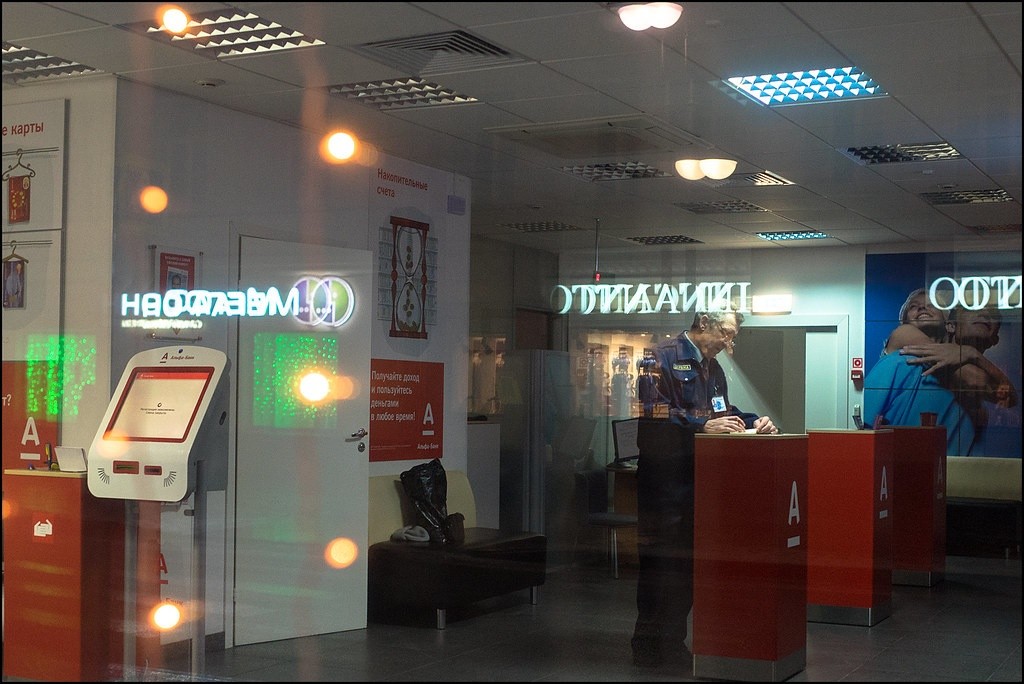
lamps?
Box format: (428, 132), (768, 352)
(675, 159), (738, 182)
(618, 3), (683, 32)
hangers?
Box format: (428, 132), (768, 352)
(2, 240), (29, 264)
(1, 148), (35, 181)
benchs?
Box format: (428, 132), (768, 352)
(367, 472), (546, 629)
(944, 455), (1023, 561)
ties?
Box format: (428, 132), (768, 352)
(702, 358), (709, 383)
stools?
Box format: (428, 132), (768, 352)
(570, 514), (638, 579)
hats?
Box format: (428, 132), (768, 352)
(391, 525), (430, 542)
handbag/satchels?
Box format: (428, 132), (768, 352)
(401, 456), (448, 542)
(442, 512), (466, 543)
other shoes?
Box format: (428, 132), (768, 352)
(660, 644), (693, 671)
(632, 652), (659, 666)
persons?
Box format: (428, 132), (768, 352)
(879, 287), (1020, 409)
(865, 286), (1004, 456)
(632, 298), (782, 668)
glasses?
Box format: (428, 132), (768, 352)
(717, 322), (736, 349)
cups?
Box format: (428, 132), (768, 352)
(920, 412), (937, 426)
